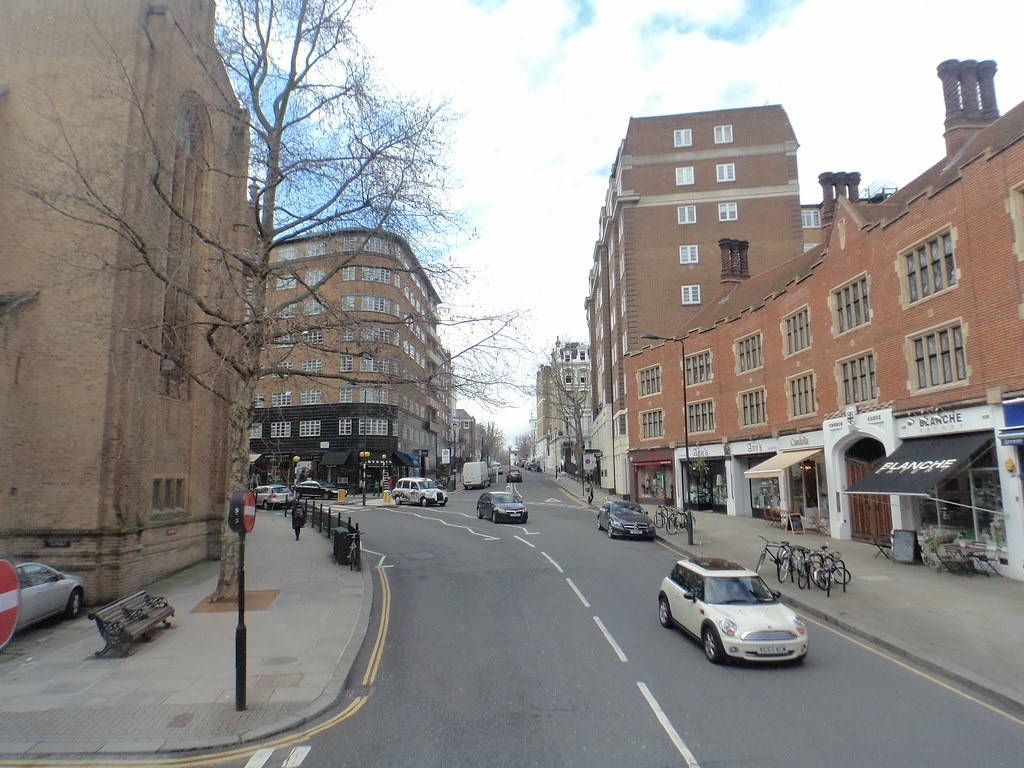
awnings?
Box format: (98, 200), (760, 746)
(250, 454), (262, 462)
(359, 451), (395, 467)
(318, 452), (351, 467)
(395, 452), (420, 466)
(744, 448), (824, 479)
(842, 428), (1024, 514)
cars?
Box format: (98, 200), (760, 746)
(659, 558), (810, 666)
(514, 458), (541, 471)
(290, 480), (348, 500)
(596, 501), (656, 540)
(504, 470), (523, 482)
(476, 491), (529, 524)
(491, 463), (503, 475)
(252, 485), (293, 510)
(392, 477), (449, 507)
(15, 561), (85, 634)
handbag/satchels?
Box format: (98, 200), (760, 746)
(585, 487), (589, 492)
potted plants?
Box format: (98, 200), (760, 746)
(689, 457), (710, 489)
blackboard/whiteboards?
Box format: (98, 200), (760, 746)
(786, 514), (804, 533)
(893, 530), (920, 562)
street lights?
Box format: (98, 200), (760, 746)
(292, 455), (300, 479)
(452, 421), (458, 490)
(359, 451), (370, 506)
(382, 454), (388, 467)
(639, 331), (694, 545)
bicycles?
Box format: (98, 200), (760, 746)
(344, 532), (365, 571)
(652, 505), (697, 535)
(755, 533), (852, 591)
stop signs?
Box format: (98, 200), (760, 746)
(243, 490), (256, 532)
(1, 558), (22, 649)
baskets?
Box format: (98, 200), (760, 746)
(833, 551), (841, 562)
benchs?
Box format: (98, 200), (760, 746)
(88, 588), (175, 658)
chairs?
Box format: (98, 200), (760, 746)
(763, 507), (788, 529)
(809, 515), (831, 537)
(871, 533), (889, 559)
(935, 541), (1004, 578)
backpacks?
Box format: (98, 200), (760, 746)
(295, 505), (303, 519)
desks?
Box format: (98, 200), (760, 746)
(962, 546), (987, 552)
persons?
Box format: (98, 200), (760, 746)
(642, 474), (650, 497)
(373, 478), (380, 497)
(652, 475), (657, 497)
(292, 501), (305, 541)
(388, 476), (393, 493)
(585, 478), (594, 505)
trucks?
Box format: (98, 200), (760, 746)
(463, 461), (492, 490)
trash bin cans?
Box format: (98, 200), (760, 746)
(448, 480), (454, 492)
(333, 527), (352, 565)
